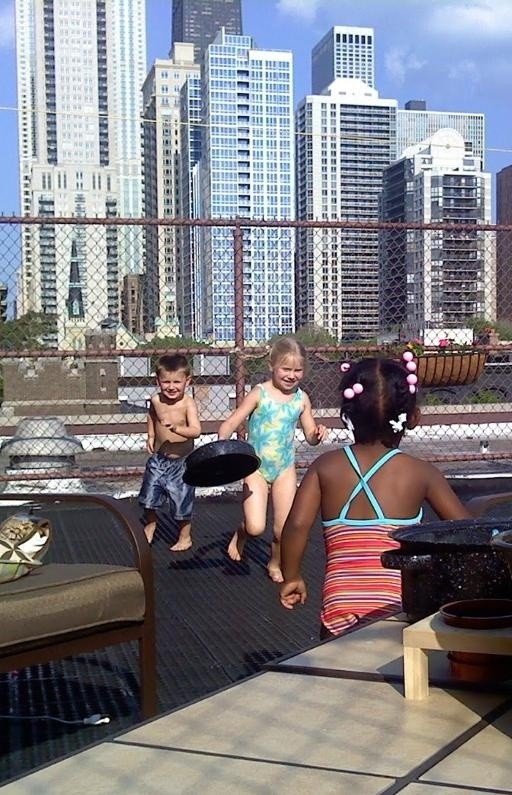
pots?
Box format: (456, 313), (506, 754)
(438, 597), (510, 688)
(381, 518), (512, 615)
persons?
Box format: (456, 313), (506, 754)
(218, 337), (327, 583)
(138, 354), (202, 552)
(280, 357), (474, 641)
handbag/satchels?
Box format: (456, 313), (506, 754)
(0, 513), (53, 583)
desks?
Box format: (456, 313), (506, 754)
(399, 612), (510, 701)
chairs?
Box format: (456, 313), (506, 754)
(1, 493), (158, 723)
